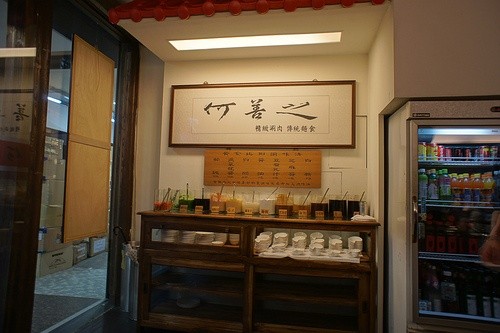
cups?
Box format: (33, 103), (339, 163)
(154, 191), (369, 219)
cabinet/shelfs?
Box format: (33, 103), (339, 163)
(137, 211), (381, 333)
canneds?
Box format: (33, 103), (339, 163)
(417, 141), (500, 161)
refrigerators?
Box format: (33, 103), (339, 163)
(382, 99), (500, 333)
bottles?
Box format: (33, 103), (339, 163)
(417, 210), (490, 254)
(418, 259), (500, 320)
(417, 169), (500, 207)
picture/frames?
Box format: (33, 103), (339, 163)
(168, 80), (355, 148)
(0, 89), (34, 179)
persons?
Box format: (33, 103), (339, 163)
(480, 210), (500, 267)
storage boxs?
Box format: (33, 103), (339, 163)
(36, 159), (110, 277)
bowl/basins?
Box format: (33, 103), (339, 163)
(215, 233), (240, 245)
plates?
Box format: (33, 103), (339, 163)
(176, 298), (200, 308)
(163, 230), (215, 245)
